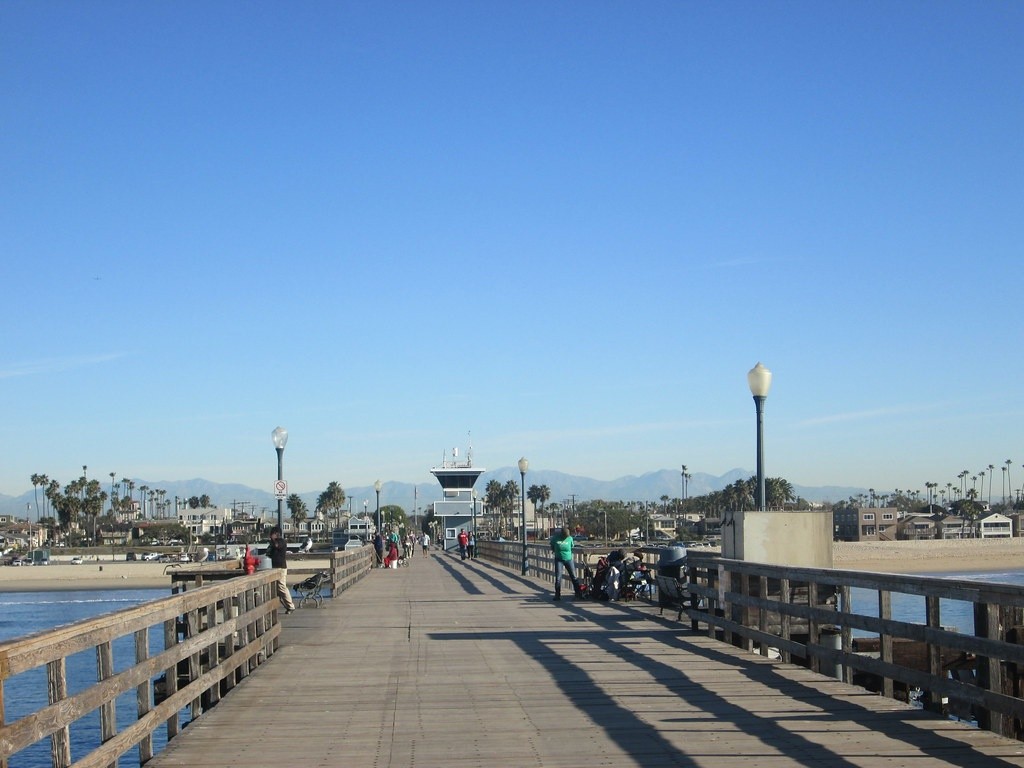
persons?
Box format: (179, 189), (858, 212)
(403, 531), (418, 559)
(389, 531), (398, 543)
(466, 531), (475, 560)
(550, 528), (582, 601)
(458, 529), (468, 560)
(372, 531), (383, 568)
(384, 543), (398, 568)
(266, 526), (296, 615)
(419, 532), (430, 558)
(595, 548), (650, 598)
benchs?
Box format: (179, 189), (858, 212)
(656, 575), (704, 620)
(294, 572), (327, 608)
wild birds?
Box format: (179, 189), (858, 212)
(192, 548), (210, 566)
(300, 537), (314, 554)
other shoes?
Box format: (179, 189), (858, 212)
(575, 591), (582, 597)
(285, 608), (295, 614)
(553, 593), (561, 600)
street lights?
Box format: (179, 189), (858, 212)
(518, 456), (530, 578)
(747, 361), (772, 511)
(363, 498), (369, 515)
(374, 479), (383, 533)
(472, 488), (478, 557)
(271, 425), (290, 537)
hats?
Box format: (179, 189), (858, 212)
(561, 529), (570, 536)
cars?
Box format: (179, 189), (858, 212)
(4, 557), (50, 565)
(126, 535), (215, 563)
(40, 539), (65, 548)
(71, 556), (83, 565)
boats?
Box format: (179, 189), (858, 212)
(153, 517), (261, 701)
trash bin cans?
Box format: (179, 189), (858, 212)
(253, 548), (273, 570)
(385, 539), (389, 551)
(657, 547), (686, 608)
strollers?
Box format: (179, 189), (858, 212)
(577, 560), (608, 600)
